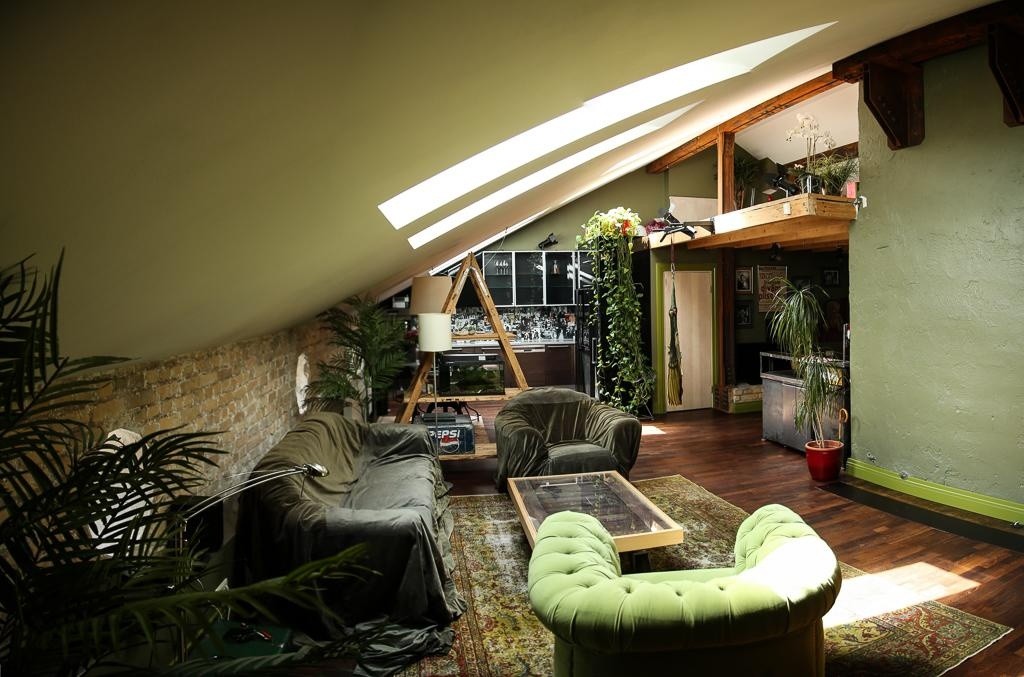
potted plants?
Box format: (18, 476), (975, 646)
(734, 158), (761, 209)
(763, 275), (851, 481)
(803, 149), (860, 197)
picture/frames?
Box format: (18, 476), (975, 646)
(820, 267), (842, 288)
(734, 300), (753, 329)
(735, 265), (753, 296)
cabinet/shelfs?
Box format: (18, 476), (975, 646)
(481, 251), (598, 308)
(441, 345), (577, 387)
(394, 252), (531, 461)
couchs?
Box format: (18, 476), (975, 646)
(493, 385), (643, 493)
(231, 409), (468, 677)
(525, 505), (842, 676)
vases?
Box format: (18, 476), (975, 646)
(795, 172), (822, 194)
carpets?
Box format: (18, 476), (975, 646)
(392, 472), (1015, 677)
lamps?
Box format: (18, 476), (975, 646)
(170, 462), (330, 664)
(417, 311), (453, 455)
(408, 269), (459, 316)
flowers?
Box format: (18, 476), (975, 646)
(783, 113), (836, 176)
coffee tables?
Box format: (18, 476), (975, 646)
(506, 469), (684, 573)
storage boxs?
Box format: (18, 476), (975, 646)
(438, 360), (505, 397)
